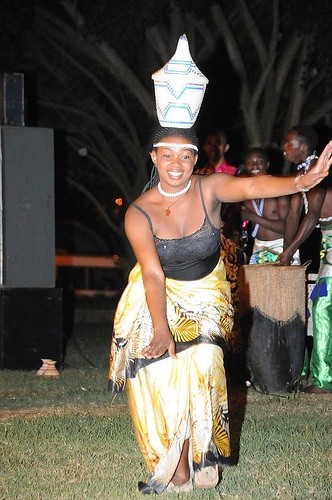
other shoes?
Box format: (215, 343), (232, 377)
(193, 463), (218, 489)
(158, 479), (193, 492)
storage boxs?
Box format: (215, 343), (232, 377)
(0, 72), (25, 126)
(0, 288), (64, 370)
(2, 127), (56, 288)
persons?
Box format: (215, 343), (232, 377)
(108, 126), (332, 493)
(193, 126), (249, 382)
(236, 148), (300, 267)
(273, 126), (332, 395)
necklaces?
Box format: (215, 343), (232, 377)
(252, 198), (264, 239)
(157, 178), (191, 216)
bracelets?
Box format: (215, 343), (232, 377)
(294, 174), (310, 192)
